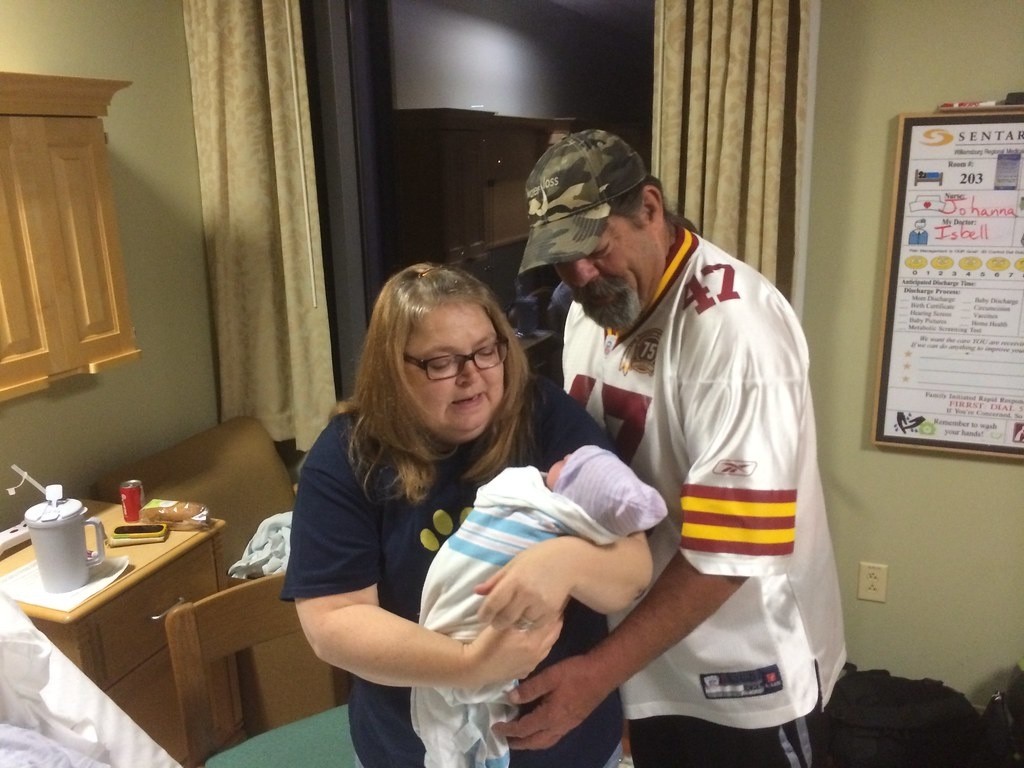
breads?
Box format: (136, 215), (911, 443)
(140, 500), (208, 530)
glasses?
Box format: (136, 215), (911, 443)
(403, 334), (509, 381)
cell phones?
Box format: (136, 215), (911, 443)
(114, 524), (167, 538)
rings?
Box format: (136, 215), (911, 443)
(517, 616), (530, 633)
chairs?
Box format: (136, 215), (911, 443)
(165, 574), (343, 768)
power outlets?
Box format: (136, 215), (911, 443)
(857, 562), (889, 603)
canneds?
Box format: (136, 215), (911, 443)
(119, 480), (145, 523)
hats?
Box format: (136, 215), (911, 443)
(554, 444), (668, 537)
(518, 129), (648, 277)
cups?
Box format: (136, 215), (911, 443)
(514, 296), (539, 338)
(25, 498), (106, 592)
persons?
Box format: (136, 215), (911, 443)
(280, 261), (625, 768)
(410, 445), (667, 768)
(475, 130), (851, 768)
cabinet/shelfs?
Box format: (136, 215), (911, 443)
(0, 71), (142, 403)
(0, 500), (248, 768)
(392, 107), (652, 285)
